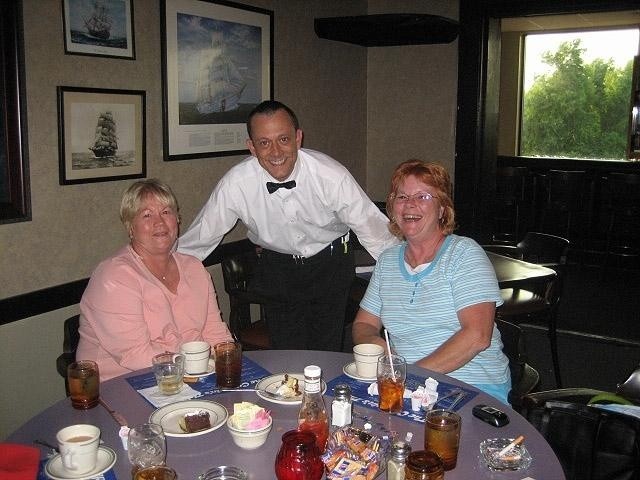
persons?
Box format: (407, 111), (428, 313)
(349, 156), (515, 413)
(168, 97), (409, 357)
(72, 177), (239, 386)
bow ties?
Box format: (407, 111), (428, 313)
(266, 180), (296, 193)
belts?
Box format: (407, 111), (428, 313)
(262, 237), (344, 266)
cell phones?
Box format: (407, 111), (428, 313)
(472, 404), (510, 427)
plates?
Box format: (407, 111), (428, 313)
(342, 361), (378, 384)
(147, 400), (228, 439)
(44, 446), (117, 480)
(255, 372), (328, 406)
(182, 358), (217, 377)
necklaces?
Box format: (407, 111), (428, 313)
(140, 256), (174, 282)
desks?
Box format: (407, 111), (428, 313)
(347, 248), (558, 290)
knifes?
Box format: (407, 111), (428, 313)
(97, 395), (129, 430)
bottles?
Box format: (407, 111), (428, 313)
(385, 441), (413, 479)
(275, 429), (323, 480)
(297, 364), (330, 453)
(405, 450), (445, 480)
(329, 381), (354, 432)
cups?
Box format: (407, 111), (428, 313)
(127, 424), (168, 469)
(133, 466), (176, 480)
(151, 352), (185, 396)
(55, 425), (101, 475)
(423, 409), (461, 470)
(65, 360), (101, 411)
(199, 465), (248, 480)
(376, 354), (407, 414)
(226, 412), (272, 451)
(352, 342), (387, 378)
(172, 340), (211, 375)
(213, 341), (243, 389)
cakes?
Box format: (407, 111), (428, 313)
(276, 375), (298, 399)
(177, 410), (211, 433)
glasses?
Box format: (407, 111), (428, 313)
(389, 191), (439, 203)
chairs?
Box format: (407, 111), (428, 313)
(475, 164), (640, 278)
(218, 250), (277, 349)
(519, 366), (640, 478)
(53, 312), (80, 399)
(493, 319), (541, 411)
(492, 230), (572, 387)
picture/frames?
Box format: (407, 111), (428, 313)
(60, 1), (138, 63)
(0, 0), (34, 226)
(54, 85), (149, 186)
(158, 1), (277, 162)
